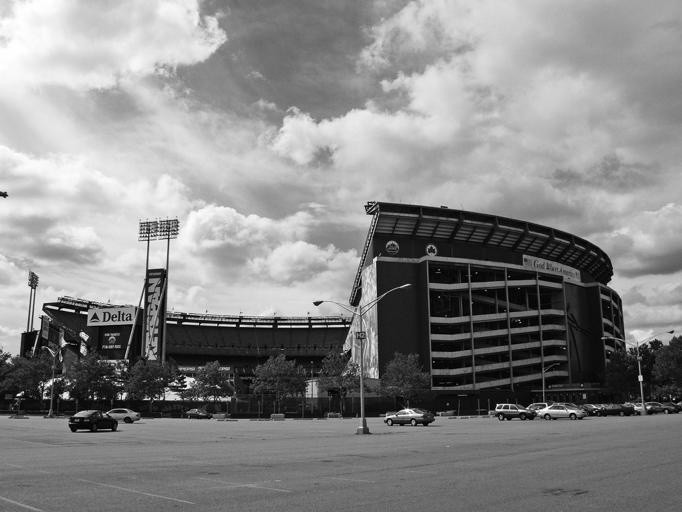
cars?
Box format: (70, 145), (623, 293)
(384, 408), (435, 426)
(186, 408), (210, 419)
(68, 408), (140, 432)
(494, 402), (682, 420)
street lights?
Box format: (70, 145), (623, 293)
(313, 283), (412, 435)
(41, 343), (70, 418)
(542, 362), (560, 403)
(20, 272), (38, 358)
(601, 329), (674, 416)
(119, 219), (179, 368)
